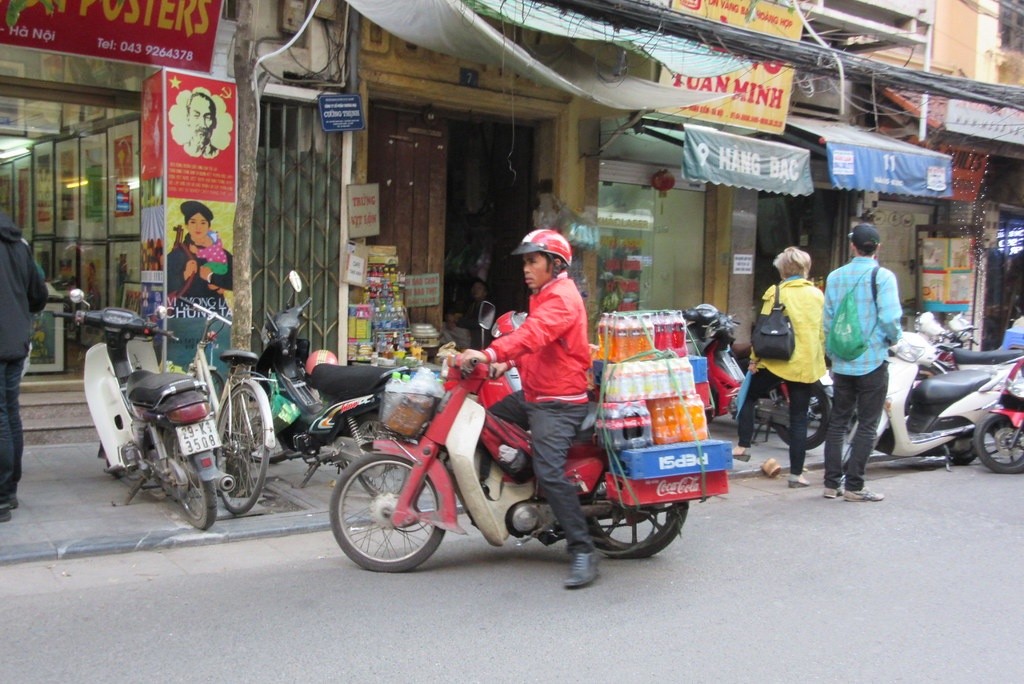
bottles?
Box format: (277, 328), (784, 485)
(349, 265), (414, 358)
(380, 371), (410, 423)
(595, 309), (707, 451)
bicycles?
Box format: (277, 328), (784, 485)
(180, 298), (278, 512)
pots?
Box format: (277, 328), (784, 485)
(411, 324), (444, 347)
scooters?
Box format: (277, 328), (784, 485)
(842, 332), (1022, 472)
(973, 344), (1024, 474)
(914, 311), (961, 342)
(944, 311), (978, 336)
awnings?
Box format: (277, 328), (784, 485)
(639, 122), (814, 197)
(781, 115), (955, 200)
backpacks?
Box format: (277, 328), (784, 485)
(827, 267), (878, 362)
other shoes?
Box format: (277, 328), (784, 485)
(823, 487), (845, 498)
(843, 489), (884, 502)
(563, 551), (599, 587)
(0, 492), (19, 520)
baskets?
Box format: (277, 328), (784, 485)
(377, 386), (441, 442)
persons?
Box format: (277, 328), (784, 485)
(0, 205), (48, 521)
(461, 228), (599, 588)
(731, 246), (825, 488)
(825, 224), (903, 502)
(447, 278), (490, 349)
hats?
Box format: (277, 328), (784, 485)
(847, 224), (880, 248)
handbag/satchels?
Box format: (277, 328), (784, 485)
(480, 407), (534, 484)
(750, 280), (796, 362)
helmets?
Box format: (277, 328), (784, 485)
(510, 229), (572, 267)
(304, 349), (339, 388)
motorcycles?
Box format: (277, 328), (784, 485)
(933, 345), (1024, 371)
(703, 306), (832, 450)
(328, 300), (690, 574)
(680, 303), (747, 380)
(52, 288), (235, 529)
(251, 271), (409, 498)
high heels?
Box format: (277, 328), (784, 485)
(788, 473), (809, 488)
(730, 447), (752, 462)
